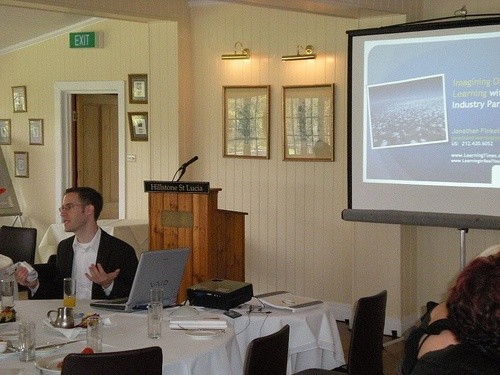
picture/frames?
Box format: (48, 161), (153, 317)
(128, 73), (149, 104)
(13, 151), (30, 179)
(127, 111), (149, 142)
(28, 117), (45, 146)
(11, 85), (28, 114)
(284, 85), (334, 160)
(223, 85), (268, 157)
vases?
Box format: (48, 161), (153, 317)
(1, 119), (11, 145)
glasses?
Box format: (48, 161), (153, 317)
(59, 203), (90, 212)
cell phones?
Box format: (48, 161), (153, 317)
(223, 310), (241, 318)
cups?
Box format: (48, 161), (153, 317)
(64, 278), (76, 308)
(19, 321), (35, 362)
(87, 316), (102, 353)
(148, 303), (163, 338)
(1, 279), (14, 308)
(150, 289), (162, 303)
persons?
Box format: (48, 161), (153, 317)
(14, 186), (139, 300)
(401, 249), (500, 374)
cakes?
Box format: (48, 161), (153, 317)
(0, 305), (16, 323)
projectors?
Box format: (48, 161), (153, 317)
(187, 278), (252, 310)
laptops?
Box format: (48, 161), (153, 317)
(90, 248), (190, 313)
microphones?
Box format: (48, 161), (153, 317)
(176, 156), (199, 170)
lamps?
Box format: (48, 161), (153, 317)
(282, 41), (317, 60)
(221, 40), (252, 61)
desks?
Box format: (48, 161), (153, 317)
(181, 290), (345, 374)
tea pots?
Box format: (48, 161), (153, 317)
(47, 306), (74, 328)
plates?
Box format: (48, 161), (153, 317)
(185, 330), (220, 340)
(34, 353), (69, 373)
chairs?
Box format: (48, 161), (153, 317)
(62, 347), (163, 369)
(0, 225), (37, 301)
(244, 325), (290, 371)
(305, 289), (389, 372)
